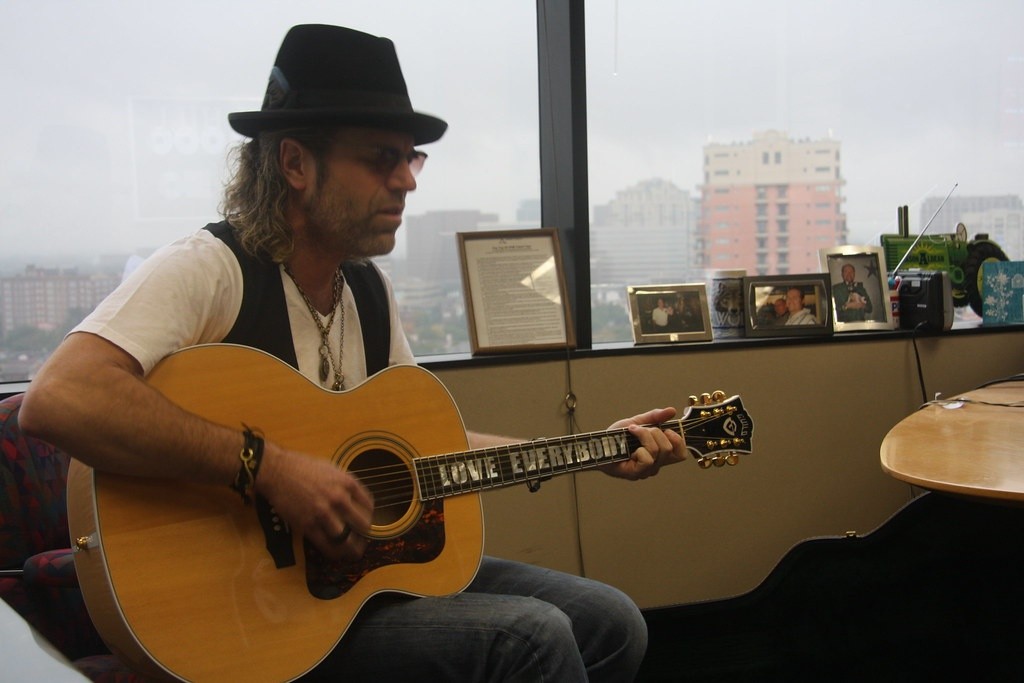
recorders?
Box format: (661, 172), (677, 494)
(892, 183), (958, 330)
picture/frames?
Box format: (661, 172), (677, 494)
(455, 227), (575, 356)
(742, 273), (834, 340)
(626, 281), (713, 347)
(817, 245), (895, 334)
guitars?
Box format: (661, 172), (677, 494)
(66, 342), (755, 683)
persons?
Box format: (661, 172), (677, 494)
(18, 25), (686, 683)
(650, 264), (872, 332)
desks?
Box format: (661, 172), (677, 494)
(879, 381), (1024, 502)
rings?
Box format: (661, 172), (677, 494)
(332, 525), (352, 544)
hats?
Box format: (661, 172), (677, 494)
(228, 24), (448, 146)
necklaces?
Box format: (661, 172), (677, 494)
(285, 259), (347, 391)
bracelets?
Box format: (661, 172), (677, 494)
(230, 428), (265, 493)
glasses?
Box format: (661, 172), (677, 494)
(315, 133), (427, 176)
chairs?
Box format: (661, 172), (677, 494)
(0, 393), (150, 683)
